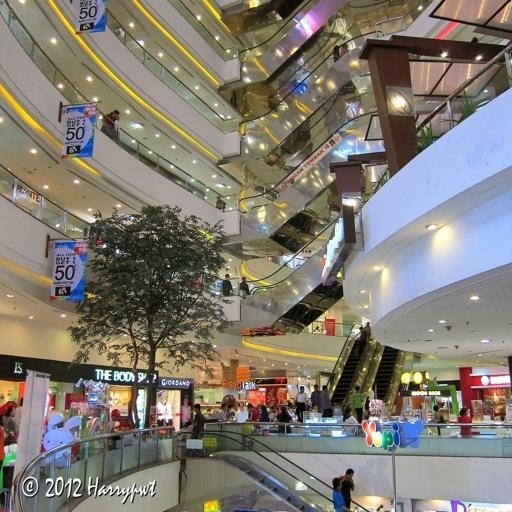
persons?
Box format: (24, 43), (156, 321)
(100, 110), (119, 140)
(240, 276), (250, 295)
(333, 46), (340, 62)
(339, 468), (355, 509)
(3, 406), (21, 445)
(343, 408), (352, 422)
(188, 403), (204, 456)
(14, 397), (23, 422)
(341, 43), (348, 55)
(458, 407), (472, 435)
(432, 405), (445, 435)
(351, 385), (364, 422)
(215, 196), (225, 211)
(332, 476), (353, 511)
(365, 321), (371, 342)
(181, 395), (192, 426)
(223, 273), (233, 296)
(114, 115), (121, 144)
(357, 327), (366, 356)
(217, 384), (332, 433)
